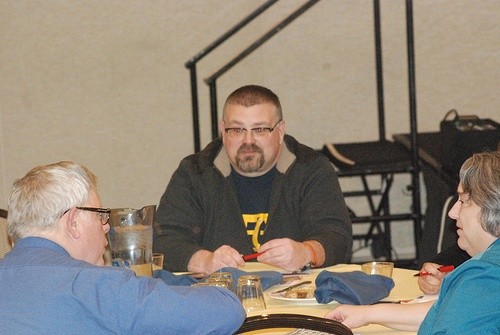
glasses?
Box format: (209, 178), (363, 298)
(223, 119), (282, 143)
(60, 207), (111, 225)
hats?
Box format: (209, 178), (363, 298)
(314, 270), (395, 306)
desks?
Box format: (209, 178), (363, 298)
(138, 261), (440, 335)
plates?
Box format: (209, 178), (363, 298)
(268, 282), (339, 304)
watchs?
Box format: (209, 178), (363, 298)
(302, 243), (317, 270)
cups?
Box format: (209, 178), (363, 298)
(236, 274), (266, 318)
(206, 277), (227, 289)
(211, 272), (234, 292)
(152, 253), (164, 273)
(361, 261), (394, 297)
(191, 282), (210, 286)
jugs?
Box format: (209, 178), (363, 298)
(106, 204), (154, 282)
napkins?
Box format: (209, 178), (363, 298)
(152, 268), (204, 287)
(314, 270), (395, 304)
(213, 267), (282, 296)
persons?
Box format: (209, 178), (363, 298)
(151, 85), (352, 276)
(325, 148), (500, 335)
(418, 244), (471, 295)
(0, 160), (247, 335)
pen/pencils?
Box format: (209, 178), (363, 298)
(242, 252), (265, 261)
(414, 265), (454, 276)
